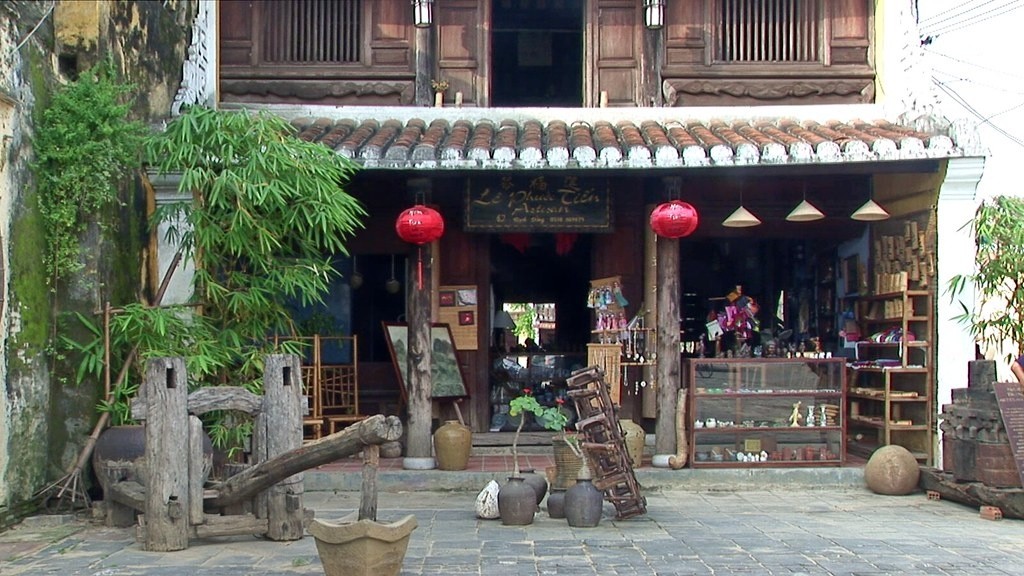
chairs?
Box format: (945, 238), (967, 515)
(265, 334), (367, 442)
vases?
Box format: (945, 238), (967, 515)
(498, 467), (602, 526)
(617, 419), (645, 468)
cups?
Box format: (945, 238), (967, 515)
(819, 447), (836, 460)
(782, 447), (796, 460)
(796, 446), (815, 460)
(771, 452), (782, 460)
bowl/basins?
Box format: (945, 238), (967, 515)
(694, 422), (703, 429)
(695, 451), (708, 460)
(696, 388), (705, 393)
(707, 388), (722, 394)
(710, 455), (723, 461)
(723, 454), (736, 461)
(706, 421), (716, 428)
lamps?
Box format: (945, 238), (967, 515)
(494, 311), (516, 346)
(785, 176), (825, 221)
(720, 177), (762, 227)
(850, 174), (890, 220)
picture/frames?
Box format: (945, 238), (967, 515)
(382, 321), (471, 404)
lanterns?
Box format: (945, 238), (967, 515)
(651, 200), (698, 240)
(397, 205), (444, 290)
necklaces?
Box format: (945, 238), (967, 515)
(624, 329), (657, 394)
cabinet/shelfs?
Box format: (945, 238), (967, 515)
(839, 290), (932, 467)
(684, 357), (847, 469)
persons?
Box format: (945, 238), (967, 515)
(1012, 353), (1024, 387)
(587, 281), (629, 344)
(509, 337), (548, 354)
(698, 333), (823, 359)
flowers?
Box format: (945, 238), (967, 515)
(542, 396), (592, 479)
(501, 389), (543, 478)
